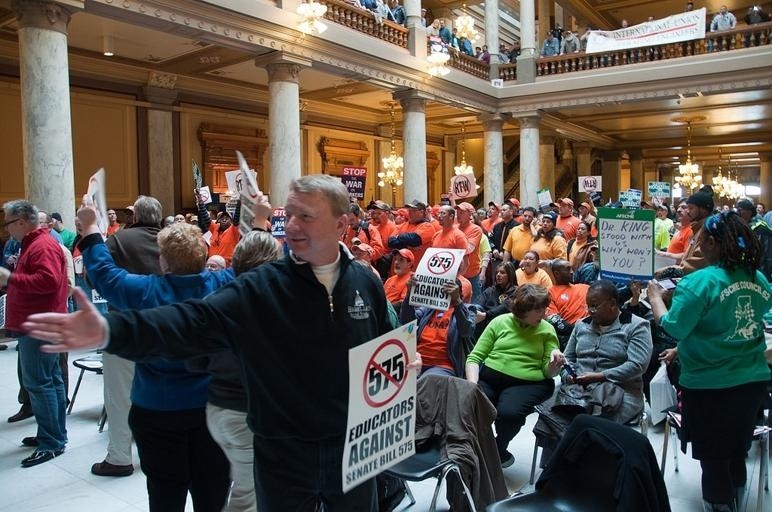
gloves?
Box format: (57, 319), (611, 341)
(554, 319), (573, 336)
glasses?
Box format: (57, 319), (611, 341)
(585, 299), (607, 313)
(2, 219), (20, 227)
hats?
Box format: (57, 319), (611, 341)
(367, 200), (426, 220)
(549, 197), (574, 208)
(454, 202), (474, 212)
(351, 243), (375, 254)
(391, 248), (414, 264)
(686, 185), (714, 212)
(489, 198), (519, 212)
(581, 192), (601, 211)
(542, 211), (557, 220)
(590, 244), (598, 251)
(641, 200), (668, 212)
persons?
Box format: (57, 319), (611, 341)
(619, 16), (663, 63)
(421, 8), (473, 55)
(679, 2), (772, 57)
(357, 0), (405, 26)
(474, 40), (520, 65)
(0, 174), (772, 512)
(541, 24), (612, 75)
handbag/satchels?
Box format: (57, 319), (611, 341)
(650, 360), (677, 427)
(377, 475), (406, 512)
(551, 382), (593, 416)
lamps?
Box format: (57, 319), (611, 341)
(449, 121), (481, 194)
(377, 100), (404, 188)
(424, 44), (451, 79)
(674, 120), (746, 201)
(293, 0), (329, 47)
(452, 3), (477, 44)
(102, 35), (115, 57)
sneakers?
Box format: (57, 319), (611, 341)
(496, 435), (509, 450)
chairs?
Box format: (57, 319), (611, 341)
(66, 352), (108, 432)
(379, 312), (772, 512)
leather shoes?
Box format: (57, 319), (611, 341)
(22, 438), (68, 446)
(8, 404), (33, 421)
(92, 461), (133, 476)
(21, 445), (64, 467)
